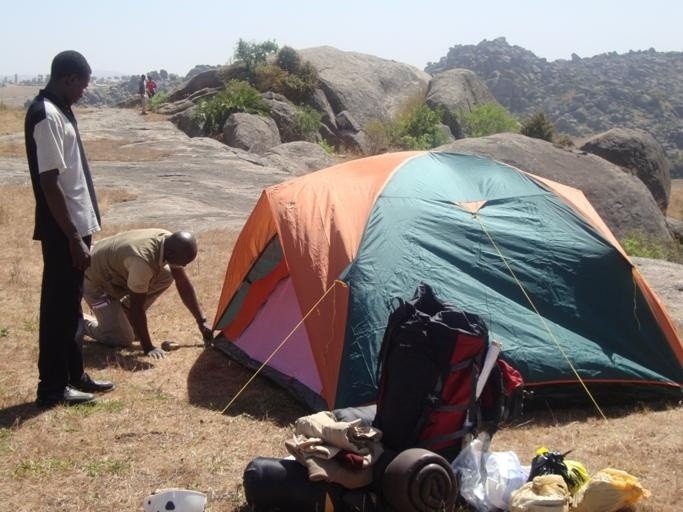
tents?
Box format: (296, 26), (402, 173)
(210, 150), (682, 417)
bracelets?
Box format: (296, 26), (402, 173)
(196, 315), (209, 324)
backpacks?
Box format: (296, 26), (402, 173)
(372, 283), (489, 463)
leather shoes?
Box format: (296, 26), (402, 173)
(66, 374), (115, 393)
(36, 380), (95, 405)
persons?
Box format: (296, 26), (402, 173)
(83, 228), (216, 360)
(146, 76), (156, 96)
(140, 76), (150, 114)
(23, 49), (116, 405)
